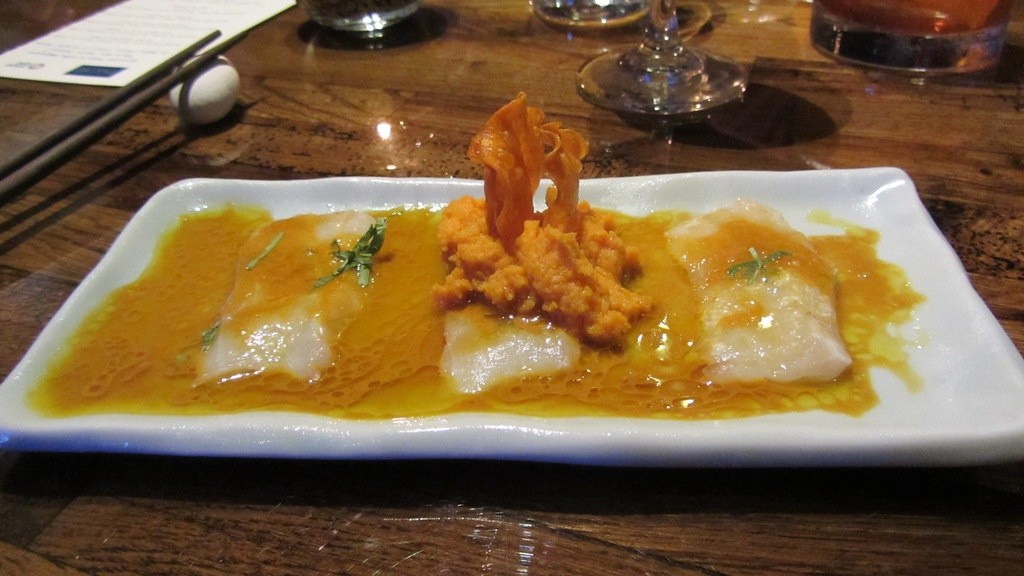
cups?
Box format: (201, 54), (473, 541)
(530, 1), (651, 24)
(300, 1), (422, 32)
(809, 1), (1009, 76)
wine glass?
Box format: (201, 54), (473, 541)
(576, 0), (748, 117)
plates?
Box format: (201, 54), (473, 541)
(2, 164), (1022, 468)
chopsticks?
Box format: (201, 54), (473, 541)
(0, 30), (246, 210)
